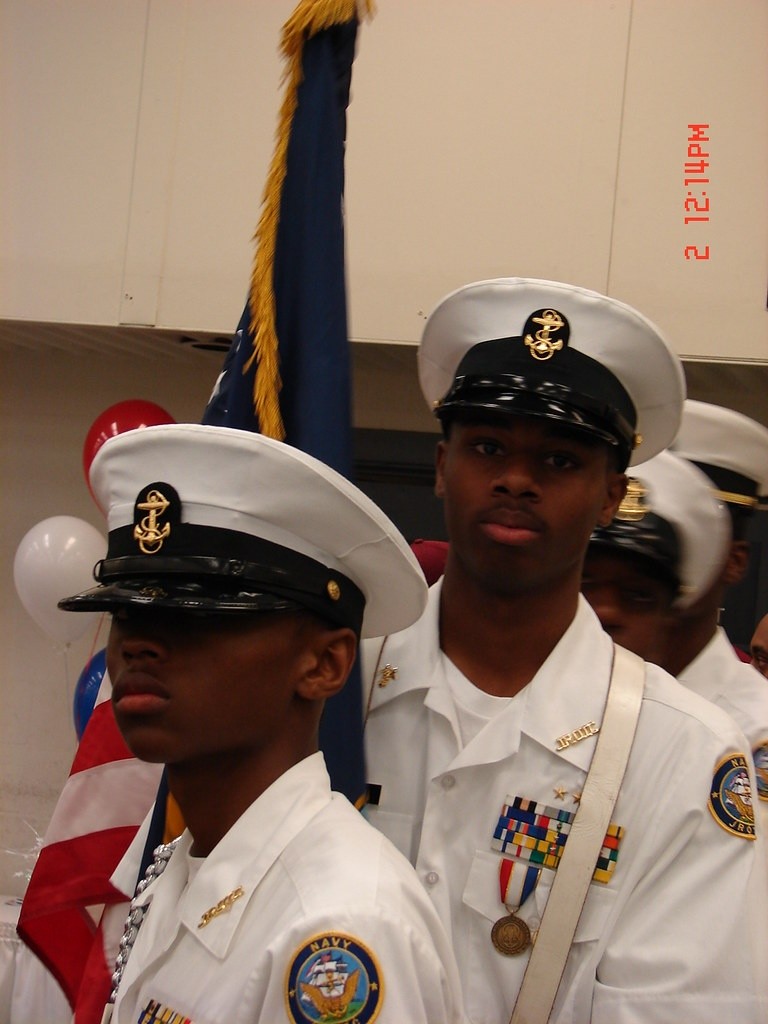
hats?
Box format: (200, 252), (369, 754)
(585, 449), (730, 614)
(670, 399), (768, 538)
(417, 278), (683, 467)
(58, 423), (428, 638)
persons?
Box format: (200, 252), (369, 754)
(90, 423), (472, 1024)
(361, 278), (768, 1024)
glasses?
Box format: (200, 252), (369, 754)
(582, 580), (668, 607)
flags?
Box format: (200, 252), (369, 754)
(17, 0), (381, 1024)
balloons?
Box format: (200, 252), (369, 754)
(84, 400), (174, 518)
(74, 647), (107, 740)
(13, 515), (109, 647)
